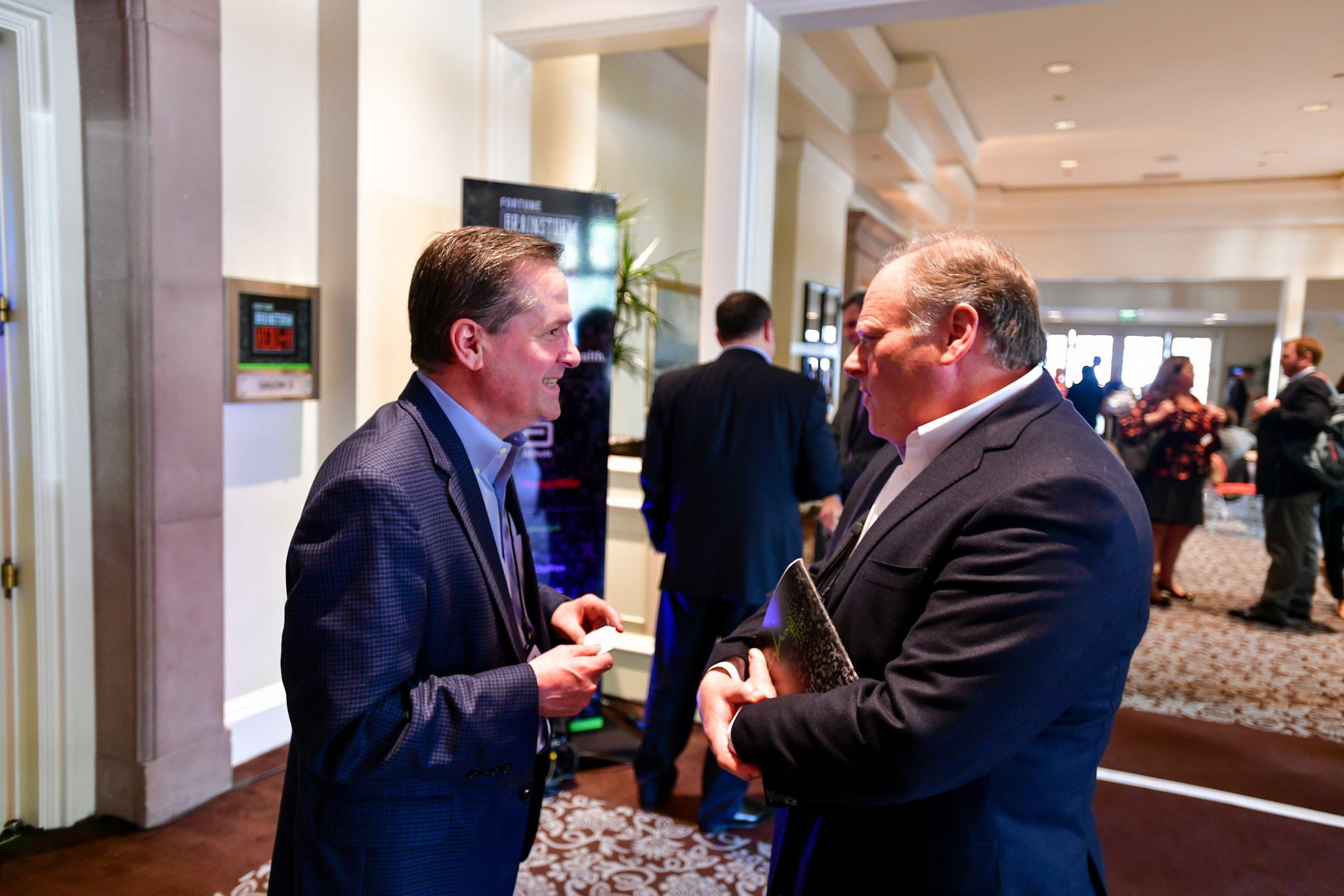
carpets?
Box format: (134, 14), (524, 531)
(209, 790), (773, 895)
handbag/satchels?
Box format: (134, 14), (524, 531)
(1116, 389), (1168, 484)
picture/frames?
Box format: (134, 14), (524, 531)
(646, 274), (700, 416)
(802, 281), (843, 412)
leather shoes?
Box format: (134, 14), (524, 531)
(699, 797), (777, 833)
(1229, 602), (1288, 628)
(640, 765), (678, 810)
(1281, 605), (1313, 627)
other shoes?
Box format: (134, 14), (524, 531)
(1150, 597), (1171, 607)
(1156, 578), (1193, 602)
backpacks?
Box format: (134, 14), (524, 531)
(1285, 374), (1344, 485)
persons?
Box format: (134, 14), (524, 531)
(266, 225), (626, 896)
(1118, 338), (1225, 606)
(1053, 366), (1123, 443)
(696, 228), (1154, 896)
(1228, 338), (1344, 628)
(1212, 365), (1257, 501)
(533, 304), (616, 601)
(629, 291), (887, 834)
